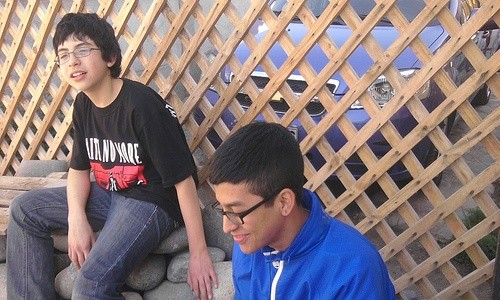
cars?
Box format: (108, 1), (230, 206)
(192, 1), (500, 190)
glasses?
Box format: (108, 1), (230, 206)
(54, 47), (104, 65)
(211, 189), (280, 225)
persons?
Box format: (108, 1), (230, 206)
(4, 12), (219, 300)
(199, 121), (397, 300)
